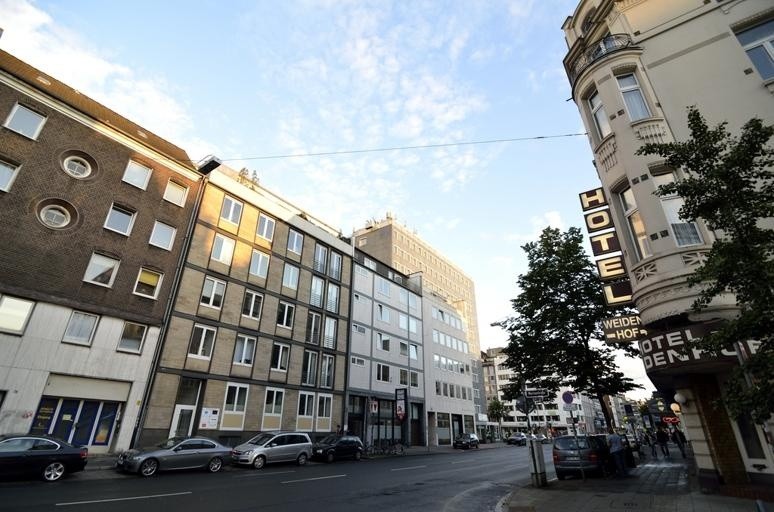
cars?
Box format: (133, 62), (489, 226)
(622, 434), (641, 452)
(453, 434), (481, 449)
(314, 433), (364, 464)
(115, 435), (235, 478)
(233, 429), (315, 469)
(508, 431), (527, 446)
(1, 433), (87, 481)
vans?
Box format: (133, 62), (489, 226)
(553, 433), (639, 479)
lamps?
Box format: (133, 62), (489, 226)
(671, 404), (682, 415)
(674, 392), (692, 405)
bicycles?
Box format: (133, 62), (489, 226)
(366, 441), (405, 460)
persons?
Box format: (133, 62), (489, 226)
(656, 427), (670, 457)
(671, 428), (687, 459)
(644, 431), (657, 457)
